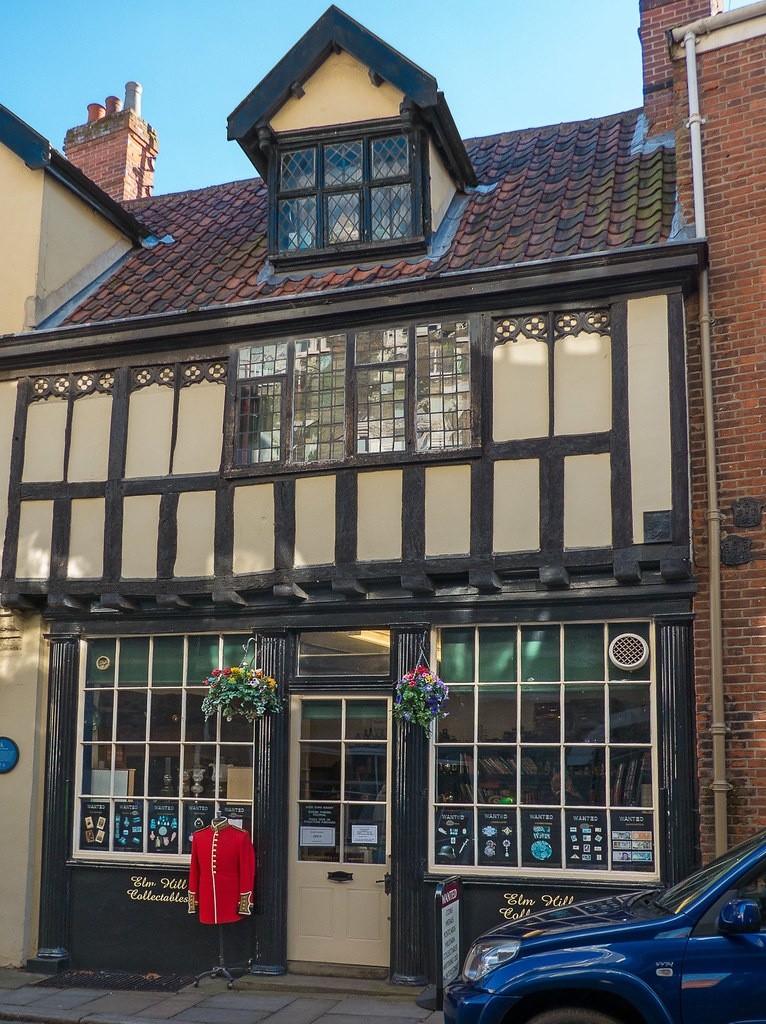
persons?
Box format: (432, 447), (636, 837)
(550, 771), (582, 806)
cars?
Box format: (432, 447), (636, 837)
(305, 742), (388, 798)
(446, 833), (766, 1024)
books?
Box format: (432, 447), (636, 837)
(465, 751), (545, 803)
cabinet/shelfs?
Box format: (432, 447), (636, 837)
(92, 768), (136, 802)
(440, 745), (560, 805)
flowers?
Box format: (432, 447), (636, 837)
(389, 664), (450, 738)
(201, 663), (283, 723)
